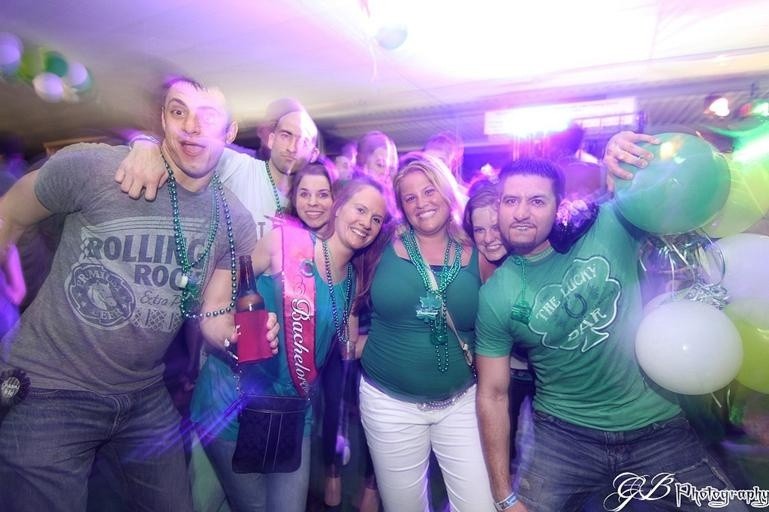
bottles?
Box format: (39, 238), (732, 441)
(228, 252), (277, 385)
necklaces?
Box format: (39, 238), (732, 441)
(0, 130), (24, 198)
(163, 155), (236, 319)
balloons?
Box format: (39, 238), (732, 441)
(0, 31), (96, 104)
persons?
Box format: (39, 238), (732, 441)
(0, 79), (280, 510)
(0, 244), (26, 339)
(114, 96), (769, 512)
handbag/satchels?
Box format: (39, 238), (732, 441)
(232, 394), (306, 474)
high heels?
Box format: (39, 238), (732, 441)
(323, 474), (343, 512)
